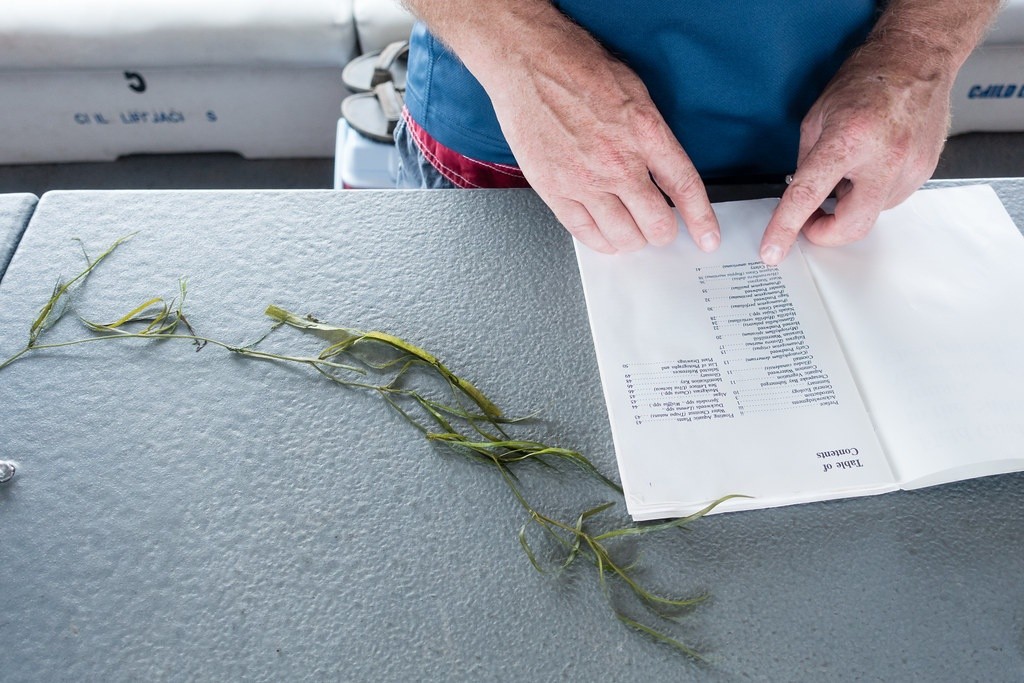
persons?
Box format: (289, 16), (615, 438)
(391, 0), (1004, 266)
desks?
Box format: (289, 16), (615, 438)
(0, 178), (1024, 683)
